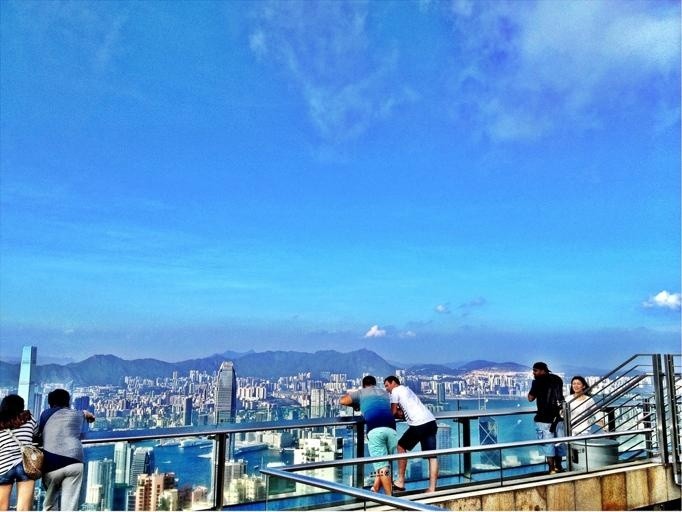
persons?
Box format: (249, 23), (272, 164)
(37, 389), (96, 511)
(527, 361), (568, 476)
(382, 375), (439, 494)
(560, 375), (605, 438)
(0, 393), (43, 510)
(339, 375), (399, 498)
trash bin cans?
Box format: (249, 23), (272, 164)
(570, 438), (619, 471)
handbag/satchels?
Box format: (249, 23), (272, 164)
(551, 400), (569, 420)
(20, 445), (45, 482)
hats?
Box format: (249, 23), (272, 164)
(533, 363), (551, 373)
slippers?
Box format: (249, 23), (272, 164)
(391, 479), (406, 493)
